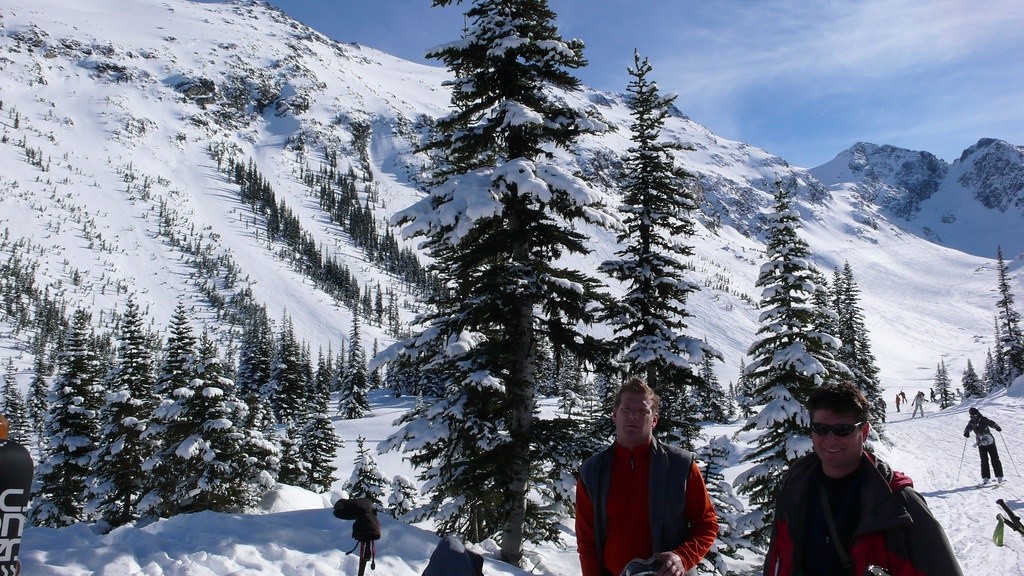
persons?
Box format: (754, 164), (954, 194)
(575, 378), (719, 576)
(764, 381), (963, 576)
(964, 407), (1003, 485)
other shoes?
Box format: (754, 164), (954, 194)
(980, 479), (988, 485)
(998, 477), (1003, 483)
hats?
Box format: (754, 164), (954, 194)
(969, 408), (981, 418)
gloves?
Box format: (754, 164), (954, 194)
(995, 426), (1002, 432)
(963, 432), (970, 438)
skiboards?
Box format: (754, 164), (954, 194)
(977, 479), (1008, 488)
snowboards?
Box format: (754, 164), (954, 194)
(0, 438), (35, 576)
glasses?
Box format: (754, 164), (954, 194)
(622, 553), (664, 576)
(808, 421), (864, 436)
